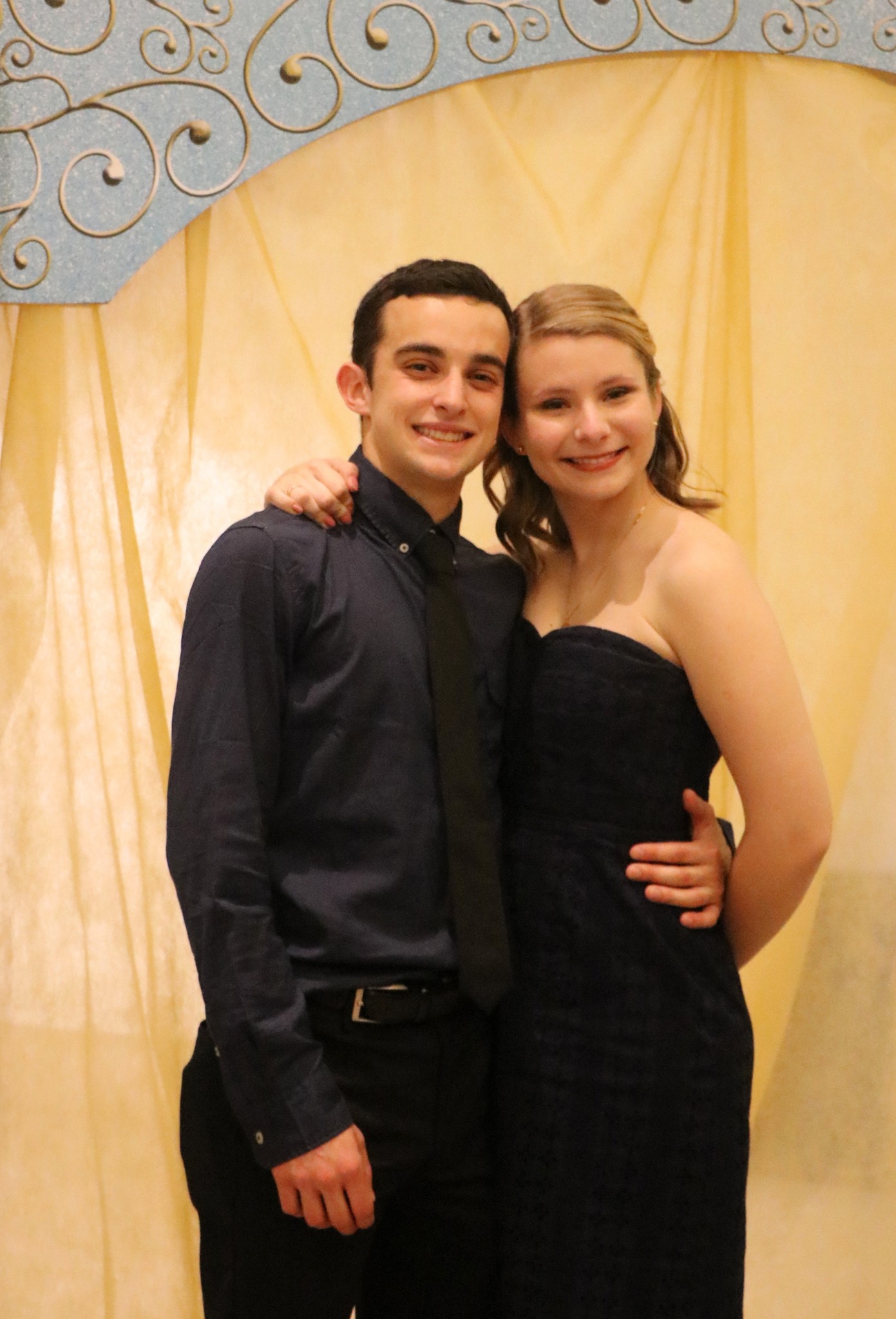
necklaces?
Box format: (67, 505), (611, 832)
(561, 490), (655, 625)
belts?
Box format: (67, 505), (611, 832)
(314, 982), (465, 1023)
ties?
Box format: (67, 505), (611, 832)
(419, 531), (512, 1010)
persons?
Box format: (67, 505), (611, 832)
(166, 259), (735, 1318)
(264, 283), (832, 1318)
